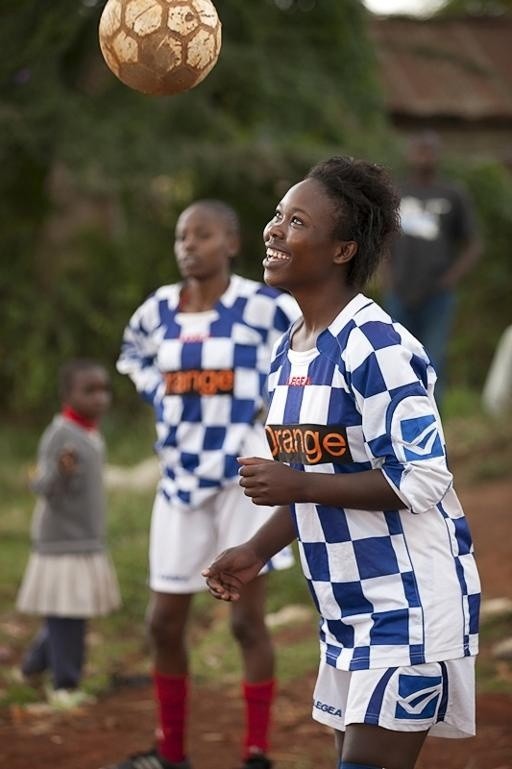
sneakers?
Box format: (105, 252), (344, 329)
(243, 749), (271, 769)
(11, 665), (99, 719)
(112, 743), (191, 769)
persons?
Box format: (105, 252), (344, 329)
(13, 358), (122, 709)
(199, 151), (484, 768)
(373, 128), (479, 418)
(113, 198), (305, 768)
(482, 315), (511, 441)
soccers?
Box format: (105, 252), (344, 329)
(99, 0), (223, 97)
